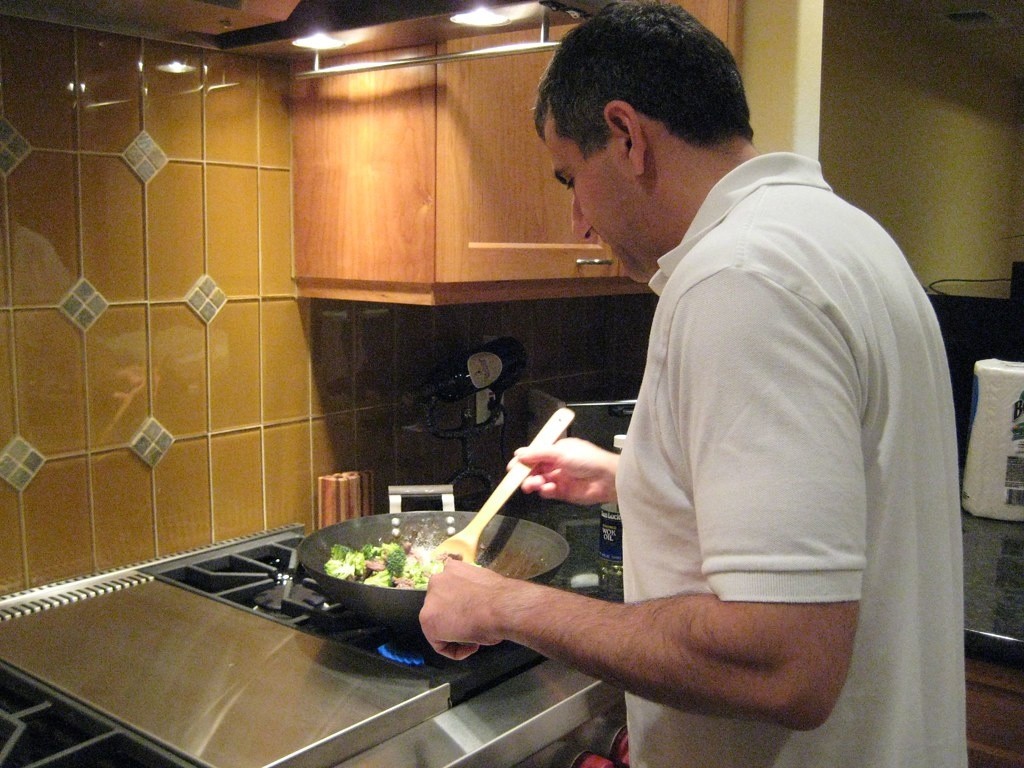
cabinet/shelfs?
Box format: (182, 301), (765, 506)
(292, 0), (743, 306)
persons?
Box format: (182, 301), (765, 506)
(419, 0), (969, 768)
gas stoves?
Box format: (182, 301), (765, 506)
(137, 518), (630, 768)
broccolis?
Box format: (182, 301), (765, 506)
(324, 541), (445, 590)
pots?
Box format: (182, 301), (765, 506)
(298, 484), (569, 646)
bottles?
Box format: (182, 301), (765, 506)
(960, 261), (1024, 522)
(597, 435), (627, 577)
